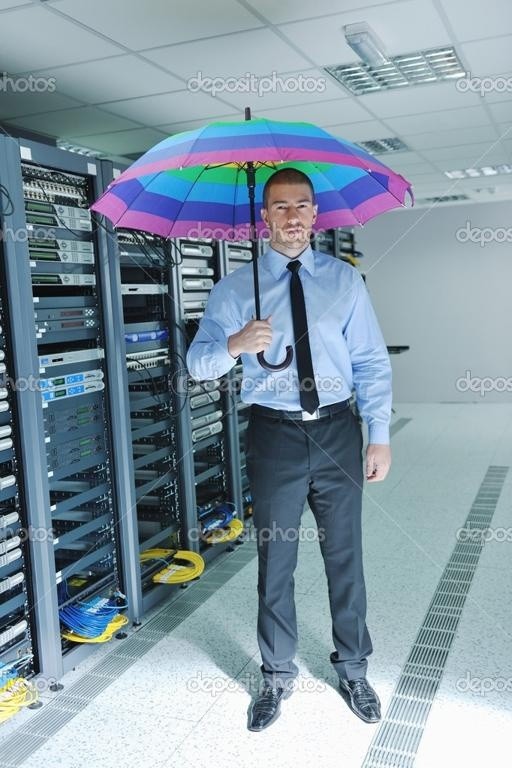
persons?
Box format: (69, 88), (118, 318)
(181, 168), (397, 734)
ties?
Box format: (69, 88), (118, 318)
(285, 260), (320, 416)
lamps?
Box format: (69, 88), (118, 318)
(340, 17), (392, 68)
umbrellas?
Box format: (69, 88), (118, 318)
(87, 103), (416, 376)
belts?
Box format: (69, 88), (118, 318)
(249, 399), (350, 422)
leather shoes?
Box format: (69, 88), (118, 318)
(338, 677), (382, 723)
(249, 685), (295, 732)
